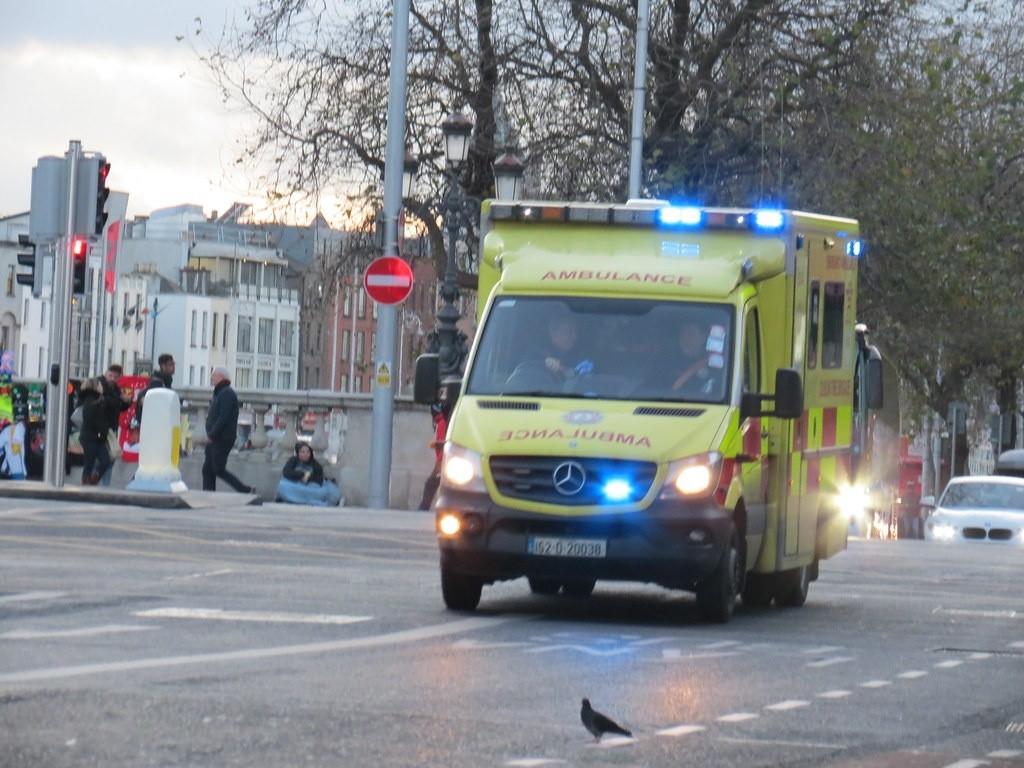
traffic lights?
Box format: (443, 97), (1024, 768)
(94, 155), (111, 234)
(15, 233), (44, 298)
(71, 235), (88, 297)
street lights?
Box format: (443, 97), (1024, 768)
(486, 141), (525, 202)
(419, 105), (479, 423)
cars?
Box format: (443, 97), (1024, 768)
(918, 476), (1024, 548)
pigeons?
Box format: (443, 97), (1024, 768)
(580, 697), (633, 744)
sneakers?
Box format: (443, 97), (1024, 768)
(248, 486), (256, 495)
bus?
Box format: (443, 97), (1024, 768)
(994, 448), (1024, 476)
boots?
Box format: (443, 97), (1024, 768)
(82, 470), (102, 485)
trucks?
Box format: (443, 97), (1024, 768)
(898, 452), (948, 510)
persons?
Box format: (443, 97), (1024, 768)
(282, 441), (324, 486)
(201, 366), (255, 494)
(78, 354), (176, 487)
(507, 311), (595, 397)
(638, 321), (724, 398)
(419, 402), (450, 511)
(901, 481), (920, 539)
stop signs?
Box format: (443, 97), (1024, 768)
(365, 257), (414, 307)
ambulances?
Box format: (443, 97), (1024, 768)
(405, 195), (864, 624)
